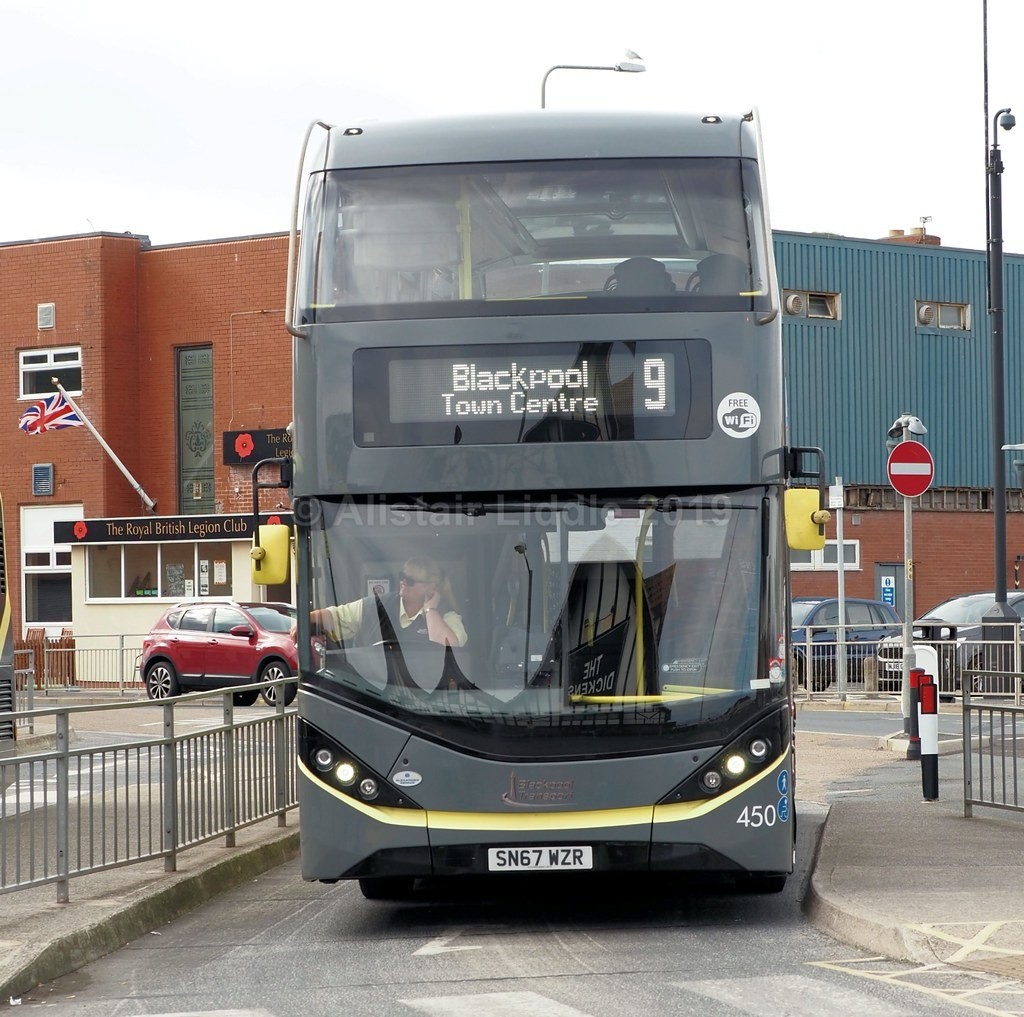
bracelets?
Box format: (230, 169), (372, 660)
(424, 608), (438, 615)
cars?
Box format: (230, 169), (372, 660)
(873, 589), (1024, 702)
(791, 597), (903, 692)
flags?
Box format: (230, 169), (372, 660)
(16, 393), (85, 436)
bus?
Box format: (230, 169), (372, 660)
(250, 106), (831, 902)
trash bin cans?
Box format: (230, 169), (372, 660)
(913, 615), (957, 703)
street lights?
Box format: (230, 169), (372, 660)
(981, 0), (1022, 698)
(541, 61), (646, 108)
(514, 542), (534, 689)
(888, 412), (927, 741)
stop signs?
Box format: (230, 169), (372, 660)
(887, 439), (935, 499)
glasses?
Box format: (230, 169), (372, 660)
(399, 571), (436, 587)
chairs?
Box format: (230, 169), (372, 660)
(602, 255), (751, 294)
(820, 606), (848, 626)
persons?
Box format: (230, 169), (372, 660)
(290, 553), (468, 691)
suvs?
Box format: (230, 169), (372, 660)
(139, 602), (326, 707)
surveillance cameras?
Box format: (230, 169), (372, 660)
(1000, 115), (1016, 130)
